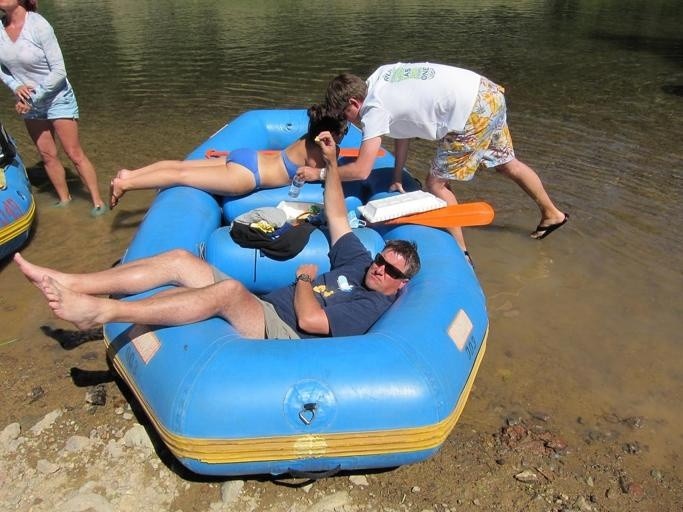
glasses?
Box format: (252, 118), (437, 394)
(341, 103), (350, 117)
(375, 253), (414, 284)
(329, 126), (350, 136)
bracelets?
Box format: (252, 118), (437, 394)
(318, 166), (325, 179)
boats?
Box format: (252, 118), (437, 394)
(101, 107), (489, 480)
(0, 122), (35, 261)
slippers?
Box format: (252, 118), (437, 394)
(531, 212), (569, 239)
(109, 170), (119, 211)
(463, 250), (473, 269)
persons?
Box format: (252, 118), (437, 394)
(0, 0), (108, 217)
(12, 131), (421, 339)
(295, 61), (569, 271)
(109, 103), (348, 210)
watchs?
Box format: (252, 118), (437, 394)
(294, 272), (312, 284)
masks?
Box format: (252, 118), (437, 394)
(347, 210), (360, 228)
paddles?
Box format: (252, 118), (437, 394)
(204, 145), (385, 159)
(288, 202), (495, 229)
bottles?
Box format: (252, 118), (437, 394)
(288, 174), (304, 199)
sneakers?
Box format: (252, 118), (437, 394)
(51, 198), (108, 217)
